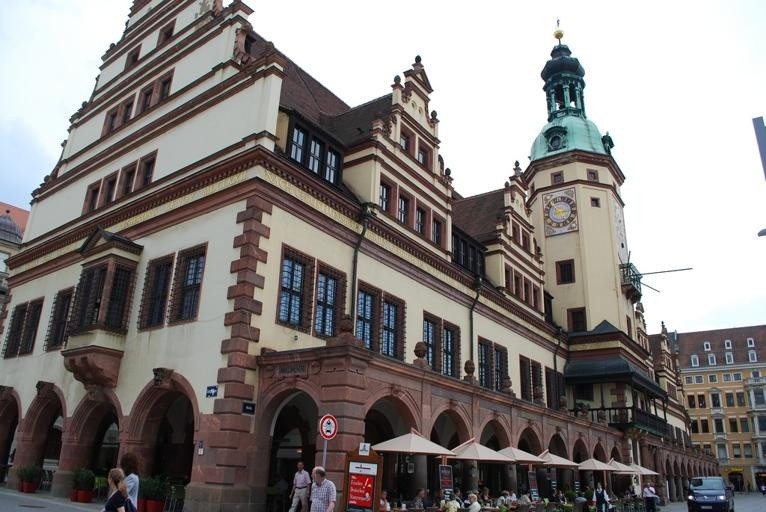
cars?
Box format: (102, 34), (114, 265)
(684, 476), (734, 512)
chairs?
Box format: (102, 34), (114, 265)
(516, 498), (557, 512)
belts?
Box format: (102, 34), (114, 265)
(295, 486), (307, 489)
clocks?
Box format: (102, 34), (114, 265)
(543, 195), (576, 229)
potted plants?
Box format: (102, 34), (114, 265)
(18, 463), (43, 493)
(135, 476), (170, 512)
(70, 467), (96, 503)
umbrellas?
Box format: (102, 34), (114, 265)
(519, 450), (580, 491)
(569, 457), (618, 473)
(370, 427), (457, 492)
(603, 458), (635, 472)
(497, 446), (545, 491)
(435, 437), (514, 492)
(612, 463), (659, 475)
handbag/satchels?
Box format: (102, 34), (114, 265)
(125, 498), (137, 512)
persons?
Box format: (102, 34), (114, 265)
(102, 467), (130, 512)
(309, 466), (337, 512)
(380, 480), (657, 512)
(286, 460), (311, 512)
(760, 483), (765, 496)
(100, 451), (140, 512)
(729, 480), (734, 498)
